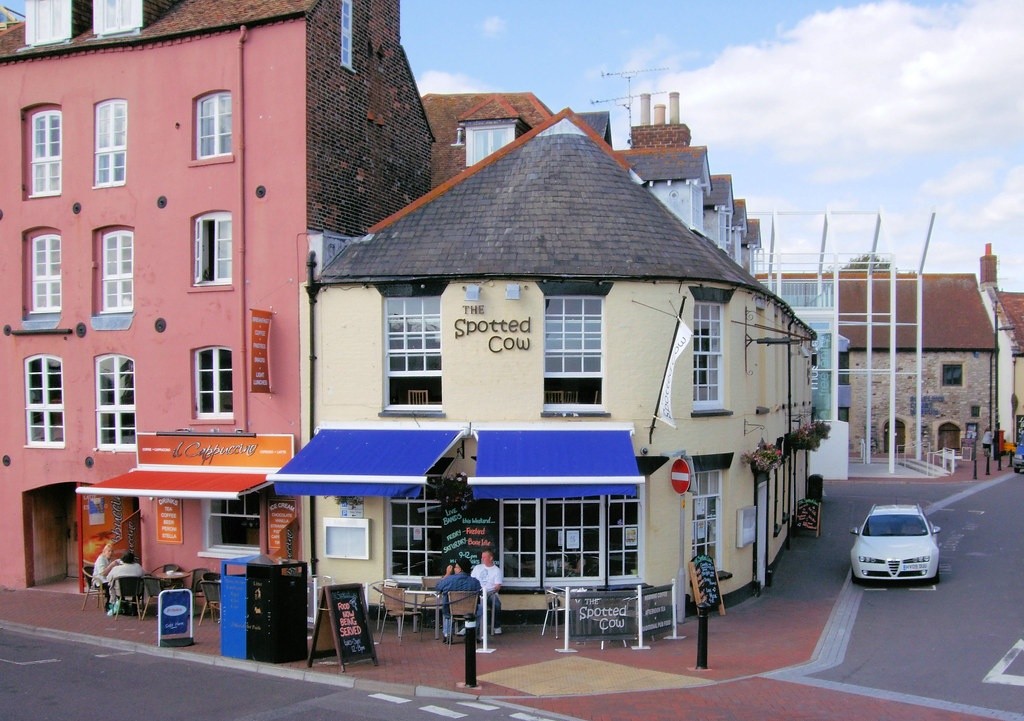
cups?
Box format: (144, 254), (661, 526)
(116, 559), (121, 564)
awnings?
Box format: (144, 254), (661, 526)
(474, 429), (645, 500)
(270, 429), (463, 498)
(75, 472), (272, 501)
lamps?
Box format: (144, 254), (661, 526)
(506, 284), (520, 300)
(464, 285), (480, 301)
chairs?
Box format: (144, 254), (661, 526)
(543, 586), (574, 639)
(901, 521), (922, 534)
(376, 577), (490, 651)
(81, 563), (221, 626)
(586, 593), (657, 650)
(408, 390), (428, 405)
(544, 390), (601, 404)
(869, 520), (891, 535)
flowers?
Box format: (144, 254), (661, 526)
(431, 470), (473, 505)
(742, 443), (783, 473)
(786, 421), (831, 449)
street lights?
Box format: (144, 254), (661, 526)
(993, 298), (1016, 461)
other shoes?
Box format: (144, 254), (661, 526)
(124, 611), (133, 615)
(134, 612), (138, 616)
(456, 628), (467, 635)
(443, 636), (452, 644)
(494, 626), (502, 634)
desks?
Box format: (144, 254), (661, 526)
(156, 572), (191, 589)
(558, 590), (638, 645)
(397, 587), (496, 640)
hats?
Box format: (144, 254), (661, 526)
(456, 557), (472, 573)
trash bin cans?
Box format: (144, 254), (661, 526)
(220, 554), (309, 664)
(808, 473), (824, 500)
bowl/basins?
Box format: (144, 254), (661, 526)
(166, 571), (174, 576)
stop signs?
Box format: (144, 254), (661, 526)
(669, 459), (690, 495)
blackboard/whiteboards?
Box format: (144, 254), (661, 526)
(311, 583), (375, 662)
(797, 498), (822, 531)
(443, 499), (501, 574)
(689, 555), (723, 608)
(641, 583), (674, 638)
(563, 590), (640, 641)
(960, 438), (977, 459)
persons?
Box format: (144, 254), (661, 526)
(434, 556), (482, 643)
(471, 550), (504, 634)
(92, 544), (146, 616)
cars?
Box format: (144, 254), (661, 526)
(849, 502), (942, 584)
(1013, 433), (1024, 473)
